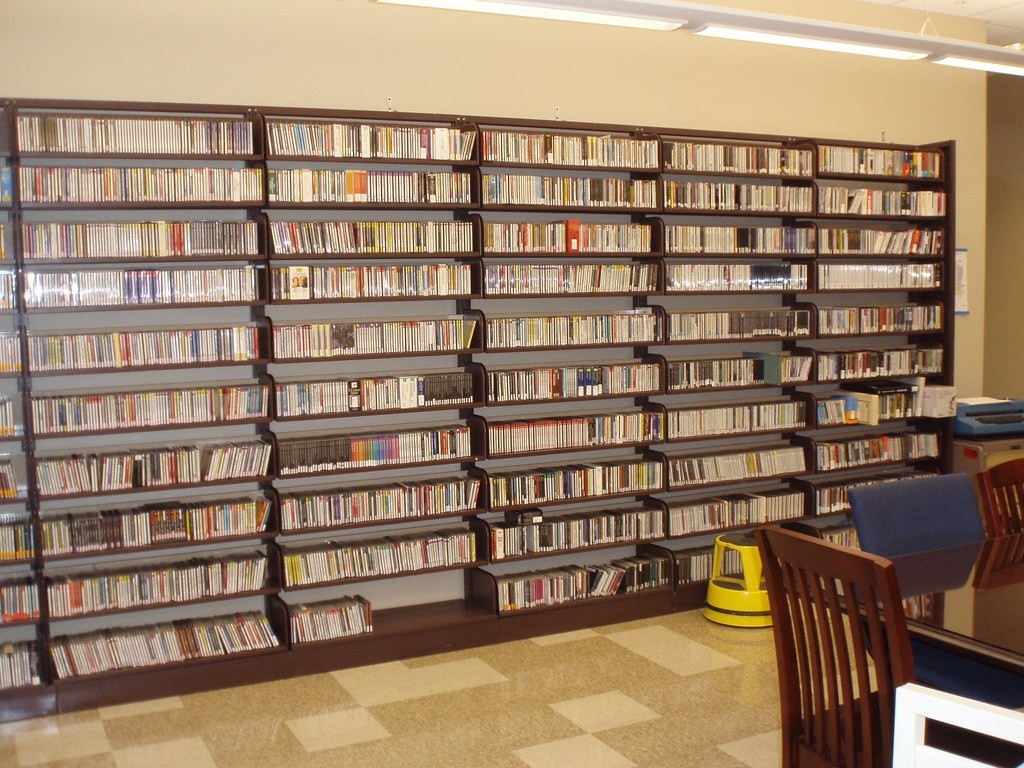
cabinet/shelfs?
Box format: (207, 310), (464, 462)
(263, 106), (497, 684)
(0, 97), (56, 727)
(12, 98), (288, 713)
(479, 110), (676, 643)
(662, 126), (818, 620)
(817, 138), (956, 549)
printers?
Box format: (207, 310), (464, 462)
(954, 396), (1024, 443)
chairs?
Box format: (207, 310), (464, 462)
(892, 682), (1024, 768)
(754, 525), (925, 768)
(976, 456), (1024, 539)
(847, 473), (987, 557)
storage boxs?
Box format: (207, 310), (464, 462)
(922, 384), (957, 418)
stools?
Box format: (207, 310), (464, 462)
(703, 532), (779, 629)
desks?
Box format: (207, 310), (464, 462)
(781, 532), (1024, 678)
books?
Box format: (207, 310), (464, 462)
(1, 96), (957, 697)
